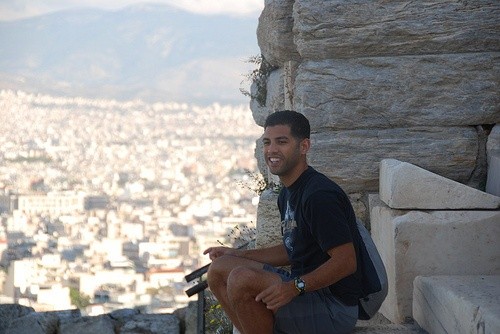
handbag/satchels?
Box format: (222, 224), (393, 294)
(356, 219), (388, 320)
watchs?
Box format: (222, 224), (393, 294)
(294, 276), (306, 296)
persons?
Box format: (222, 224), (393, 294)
(203, 110), (381, 334)
